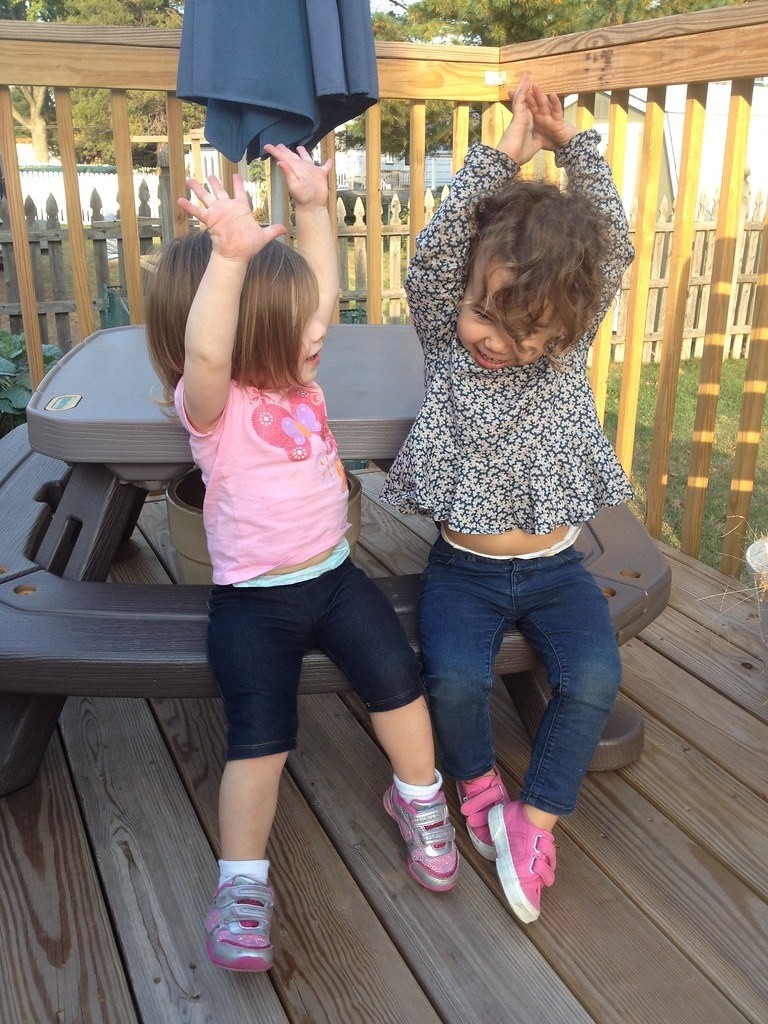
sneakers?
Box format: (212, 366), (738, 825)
(487, 800), (556, 924)
(207, 875), (275, 972)
(456, 764), (511, 862)
(383, 781), (460, 891)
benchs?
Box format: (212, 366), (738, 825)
(0, 420), (673, 701)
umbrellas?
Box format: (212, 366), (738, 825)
(176, 1), (381, 249)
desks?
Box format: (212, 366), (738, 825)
(0, 326), (647, 808)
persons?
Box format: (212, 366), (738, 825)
(384, 82), (639, 926)
(143, 139), (459, 974)
